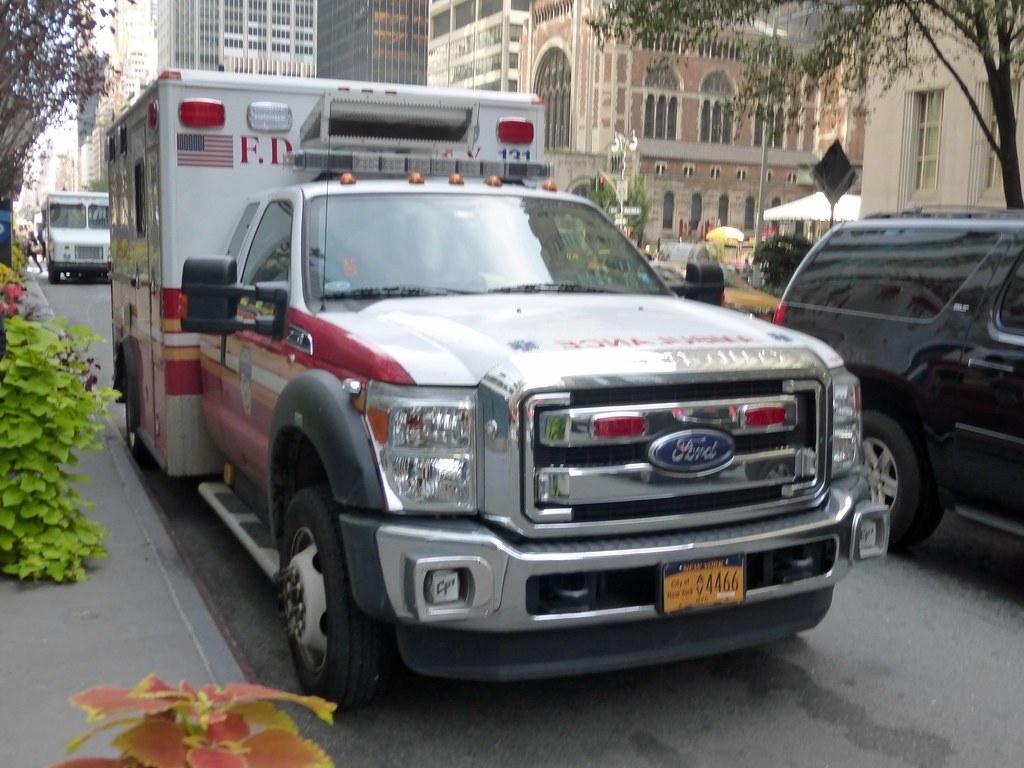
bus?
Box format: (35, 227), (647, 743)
(40, 190), (112, 283)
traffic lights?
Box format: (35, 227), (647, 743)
(596, 175), (605, 195)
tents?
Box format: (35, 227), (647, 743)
(756, 190), (876, 224)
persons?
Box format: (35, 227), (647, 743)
(25, 232), (43, 275)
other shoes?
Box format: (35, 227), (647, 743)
(39, 271), (43, 274)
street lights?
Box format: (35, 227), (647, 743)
(608, 126), (640, 230)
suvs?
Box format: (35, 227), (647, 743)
(769, 204), (1024, 555)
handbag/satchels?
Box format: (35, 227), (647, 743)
(30, 244), (43, 255)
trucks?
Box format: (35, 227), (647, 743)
(103, 69), (888, 722)
